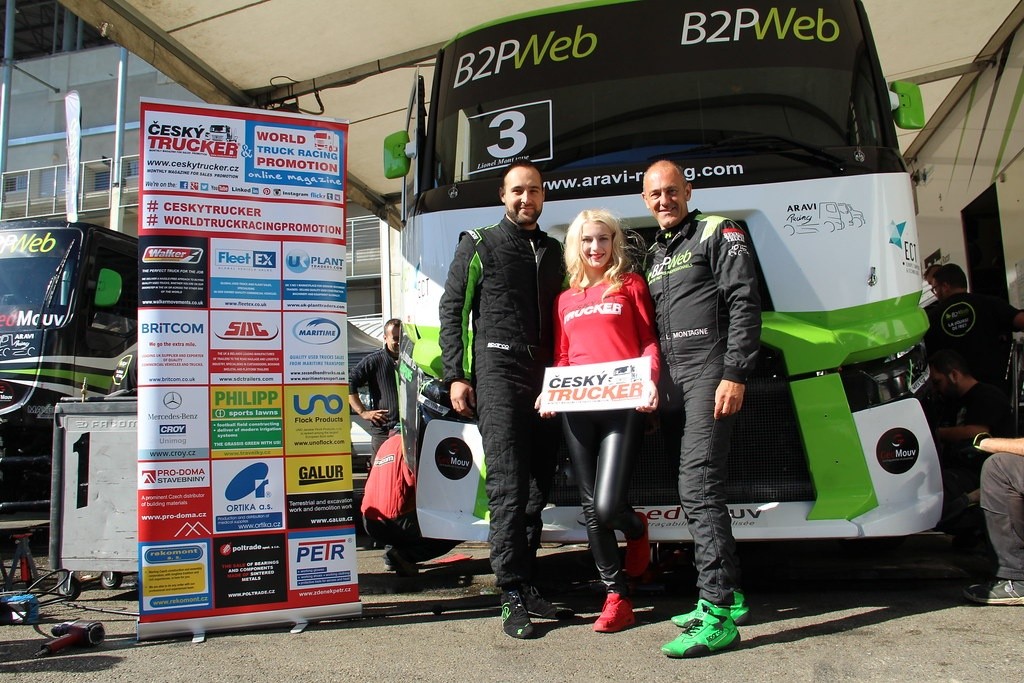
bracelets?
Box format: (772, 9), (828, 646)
(973, 432), (992, 456)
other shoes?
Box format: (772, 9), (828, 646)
(951, 533), (978, 551)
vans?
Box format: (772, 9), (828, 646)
(348, 317), (381, 524)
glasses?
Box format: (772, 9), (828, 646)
(932, 285), (938, 296)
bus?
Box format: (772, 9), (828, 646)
(1, 219), (139, 517)
(382, 0), (945, 539)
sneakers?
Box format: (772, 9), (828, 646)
(381, 546), (395, 565)
(624, 511), (650, 576)
(501, 588), (534, 640)
(672, 589), (752, 628)
(661, 598), (741, 659)
(520, 583), (575, 620)
(384, 551), (418, 576)
(965, 577), (1024, 607)
(592, 593), (636, 632)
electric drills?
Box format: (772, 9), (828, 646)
(35, 620), (105, 659)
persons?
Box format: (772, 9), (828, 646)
(436, 157), (577, 638)
(926, 348), (1016, 554)
(961, 432), (1024, 606)
(349, 317), (400, 469)
(534, 207), (664, 633)
(629, 160), (762, 659)
(923, 263), (1024, 388)
(359, 426), (467, 580)
